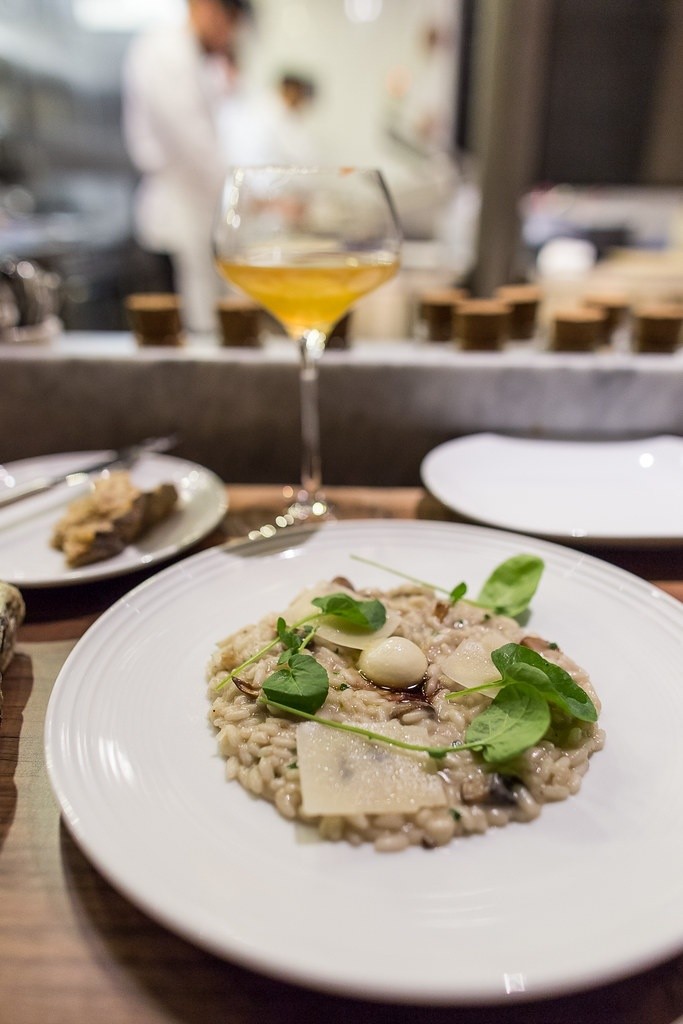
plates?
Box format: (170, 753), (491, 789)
(420, 429), (683, 552)
(0, 449), (230, 586)
(43, 517), (683, 1004)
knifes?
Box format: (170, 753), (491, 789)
(0, 428), (185, 514)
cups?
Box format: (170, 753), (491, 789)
(218, 297), (264, 349)
(125, 295), (183, 346)
(418, 285), (683, 356)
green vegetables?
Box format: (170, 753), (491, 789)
(216, 550), (598, 764)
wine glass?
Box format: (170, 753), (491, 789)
(209, 164), (405, 541)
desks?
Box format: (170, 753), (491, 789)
(1, 483), (683, 1024)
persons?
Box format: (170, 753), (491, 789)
(236, 67), (321, 167)
(118, 0), (256, 349)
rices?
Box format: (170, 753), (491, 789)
(205, 587), (605, 849)
